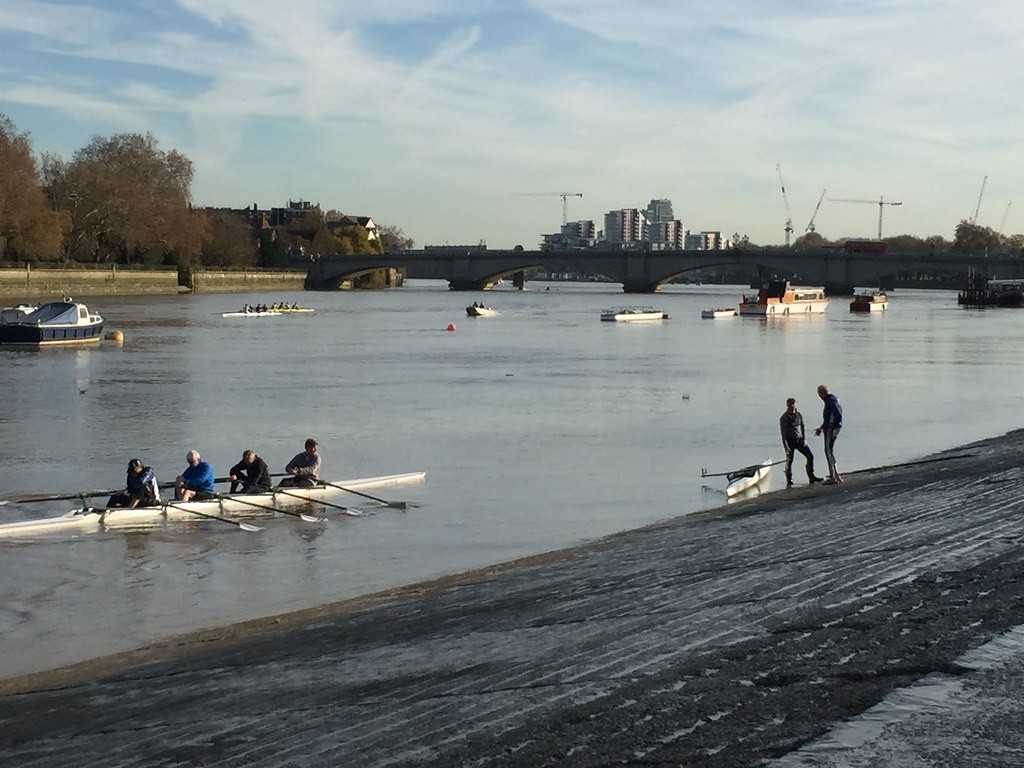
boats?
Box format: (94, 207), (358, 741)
(725, 456), (773, 497)
(466, 305), (502, 317)
(849, 286), (890, 313)
(739, 274), (831, 316)
(701, 307), (736, 318)
(600, 305), (663, 322)
(263, 307), (316, 313)
(985, 274), (1024, 308)
(0, 293), (107, 347)
(0, 471), (428, 539)
(222, 311), (282, 317)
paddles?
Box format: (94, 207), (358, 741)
(0, 489), (120, 506)
(158, 481), (175, 489)
(123, 490), (267, 532)
(238, 479), (366, 516)
(270, 471), (291, 477)
(214, 477), (233, 483)
(295, 474), (405, 508)
(180, 485), (329, 523)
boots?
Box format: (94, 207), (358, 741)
(805, 465), (824, 484)
(785, 471), (794, 485)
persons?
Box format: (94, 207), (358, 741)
(473, 302), (478, 307)
(480, 302), (484, 308)
(106, 458), (162, 508)
(175, 450), (214, 501)
(243, 301), (299, 313)
(229, 450), (272, 493)
(812, 385), (843, 485)
(780, 398), (824, 488)
(278, 438), (321, 487)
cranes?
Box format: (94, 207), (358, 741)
(967, 175), (989, 226)
(826, 195), (902, 240)
(804, 188), (827, 233)
(511, 190), (584, 226)
(775, 162), (794, 249)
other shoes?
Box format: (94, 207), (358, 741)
(824, 480), (837, 484)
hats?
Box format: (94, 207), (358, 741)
(127, 459), (142, 473)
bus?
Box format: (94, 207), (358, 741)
(844, 240), (888, 255)
